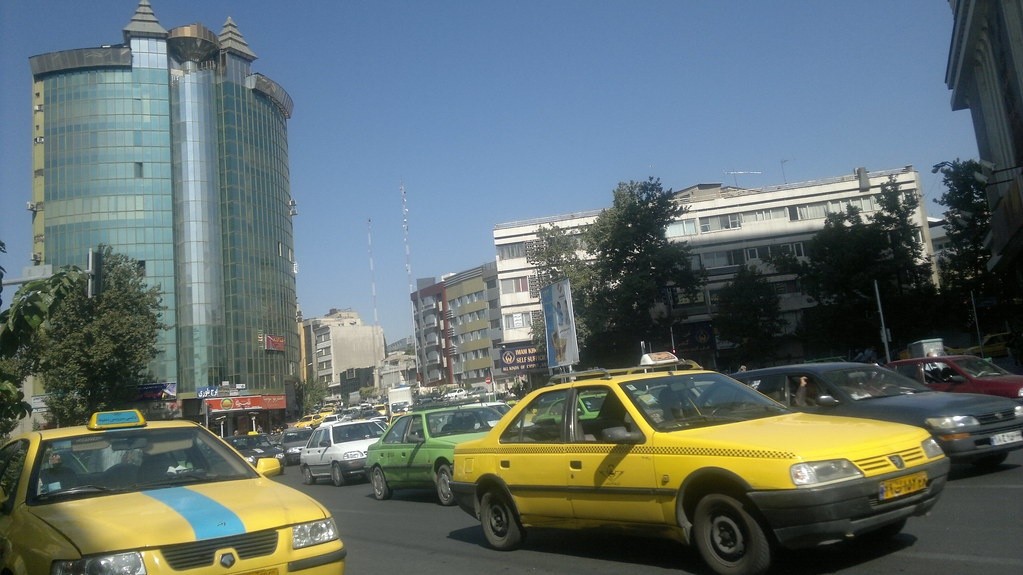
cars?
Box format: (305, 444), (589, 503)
(862, 354), (1023, 407)
(451, 347), (953, 575)
(1, 410), (348, 575)
(226, 356), (675, 511)
(688, 360), (1023, 473)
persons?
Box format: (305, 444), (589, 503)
(550, 299), (572, 327)
(794, 374), (823, 401)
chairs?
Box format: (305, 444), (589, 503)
(40, 450), (182, 499)
(391, 416), (468, 445)
(513, 391), (718, 448)
